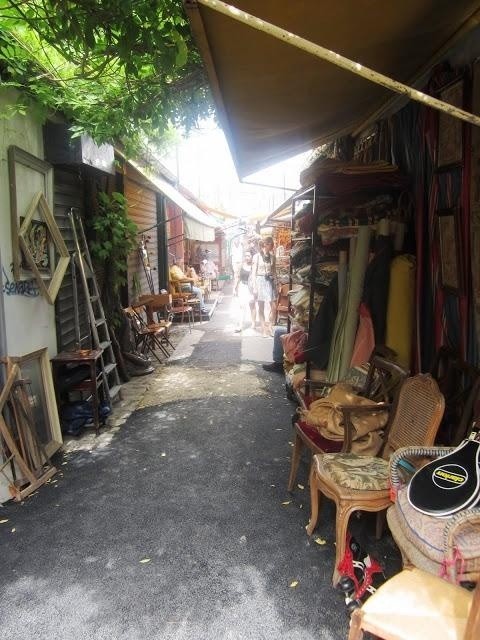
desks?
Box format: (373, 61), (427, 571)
(51, 347), (112, 437)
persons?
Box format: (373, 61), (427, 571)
(171, 257), (209, 314)
(187, 264), (200, 283)
(233, 238), (277, 338)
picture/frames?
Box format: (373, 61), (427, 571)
(17, 214), (52, 272)
(10, 346), (65, 466)
(433, 205), (466, 294)
(7, 143), (59, 284)
(19, 191), (72, 310)
(432, 65), (470, 175)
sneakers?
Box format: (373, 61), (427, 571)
(262, 363), (284, 372)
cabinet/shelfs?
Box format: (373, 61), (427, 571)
(288, 176), (400, 400)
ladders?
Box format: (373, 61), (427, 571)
(68, 207), (124, 414)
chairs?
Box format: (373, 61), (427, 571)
(285, 347), (416, 496)
(342, 558), (480, 639)
(303, 371), (445, 585)
(275, 283), (292, 328)
(380, 417), (480, 585)
(123, 279), (202, 364)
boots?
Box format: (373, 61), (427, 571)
(338, 532), (386, 615)
